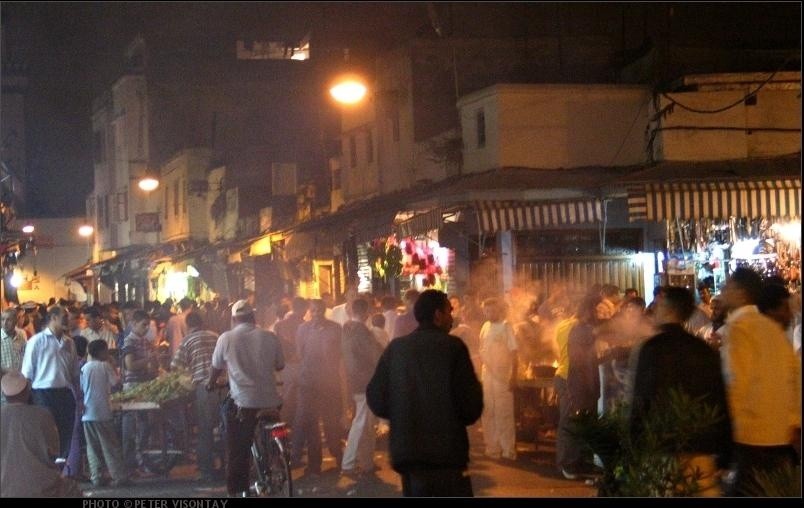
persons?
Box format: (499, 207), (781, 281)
(0, 262), (803, 497)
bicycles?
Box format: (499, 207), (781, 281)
(204, 375), (294, 497)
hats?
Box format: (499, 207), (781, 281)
(230, 299), (257, 317)
(1, 370), (27, 398)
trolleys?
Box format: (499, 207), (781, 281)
(107, 380), (196, 477)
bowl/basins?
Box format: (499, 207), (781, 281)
(531, 366), (557, 378)
